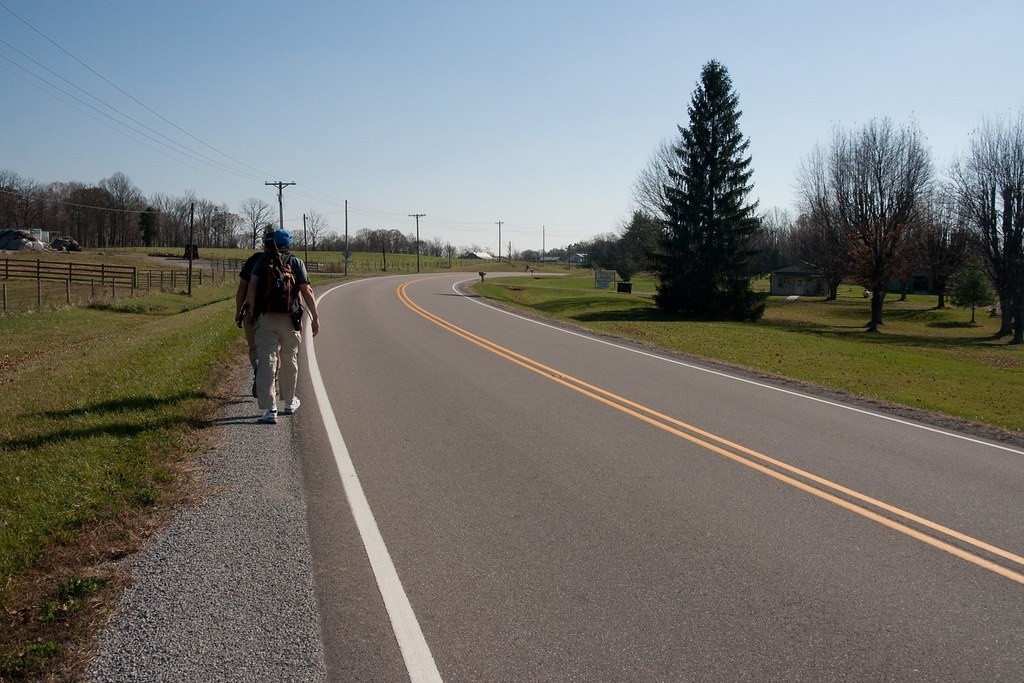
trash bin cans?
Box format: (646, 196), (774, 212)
(617, 281), (632, 293)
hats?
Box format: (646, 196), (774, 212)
(262, 224), (279, 241)
(272, 229), (293, 249)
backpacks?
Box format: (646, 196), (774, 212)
(260, 252), (293, 313)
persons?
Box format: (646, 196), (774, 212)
(235, 228), (320, 424)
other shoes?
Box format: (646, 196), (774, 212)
(252, 377), (258, 398)
(257, 408), (277, 424)
(284, 396), (300, 414)
